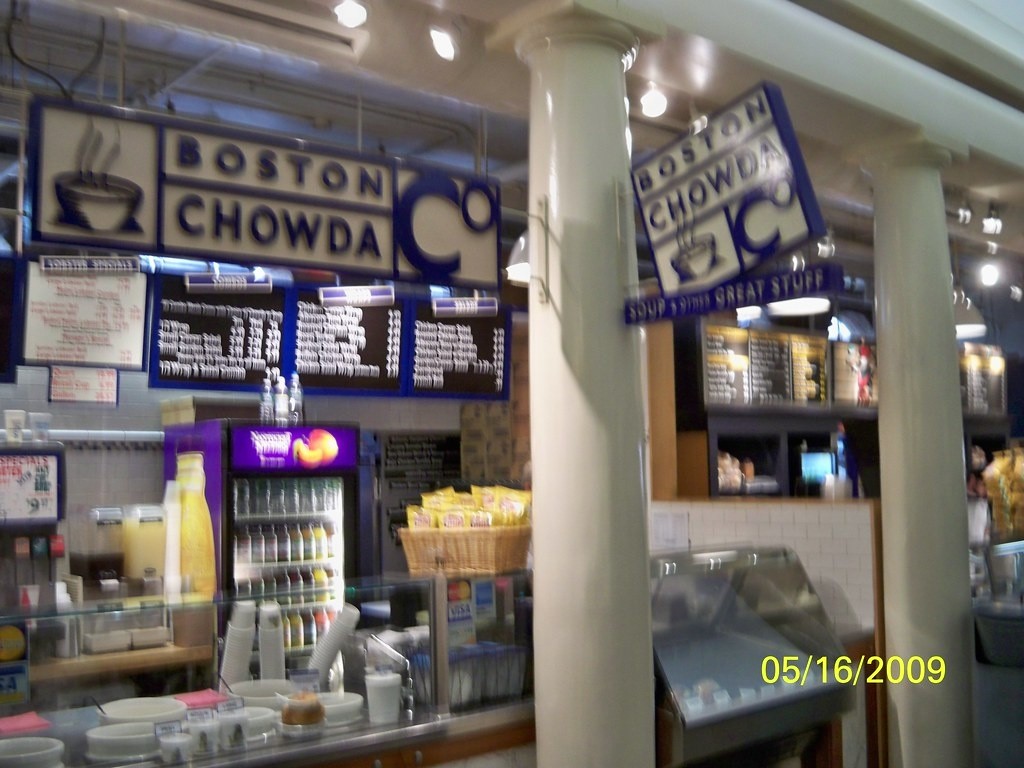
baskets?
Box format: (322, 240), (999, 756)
(397, 527), (532, 577)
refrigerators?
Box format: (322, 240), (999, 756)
(163, 418), (384, 687)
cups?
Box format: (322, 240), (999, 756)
(366, 674), (402, 723)
(159, 733), (190, 764)
(219, 713), (246, 750)
(308, 603), (360, 685)
(29, 413), (52, 442)
(219, 603), (256, 712)
(257, 602), (285, 680)
(4, 410), (26, 443)
(190, 720), (217, 755)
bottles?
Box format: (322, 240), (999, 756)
(176, 451), (216, 604)
(237, 565), (335, 605)
(234, 476), (342, 515)
(253, 607), (337, 650)
(235, 521), (335, 562)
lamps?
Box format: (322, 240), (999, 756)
(429, 15), (478, 63)
(948, 239), (986, 340)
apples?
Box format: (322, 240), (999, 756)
(282, 692), (326, 726)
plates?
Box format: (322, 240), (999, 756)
(85, 750), (159, 763)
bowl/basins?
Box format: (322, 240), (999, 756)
(0, 737), (65, 768)
(227, 679), (297, 710)
(317, 692), (364, 723)
(86, 722), (155, 754)
(245, 706), (275, 734)
(97, 697), (187, 747)
